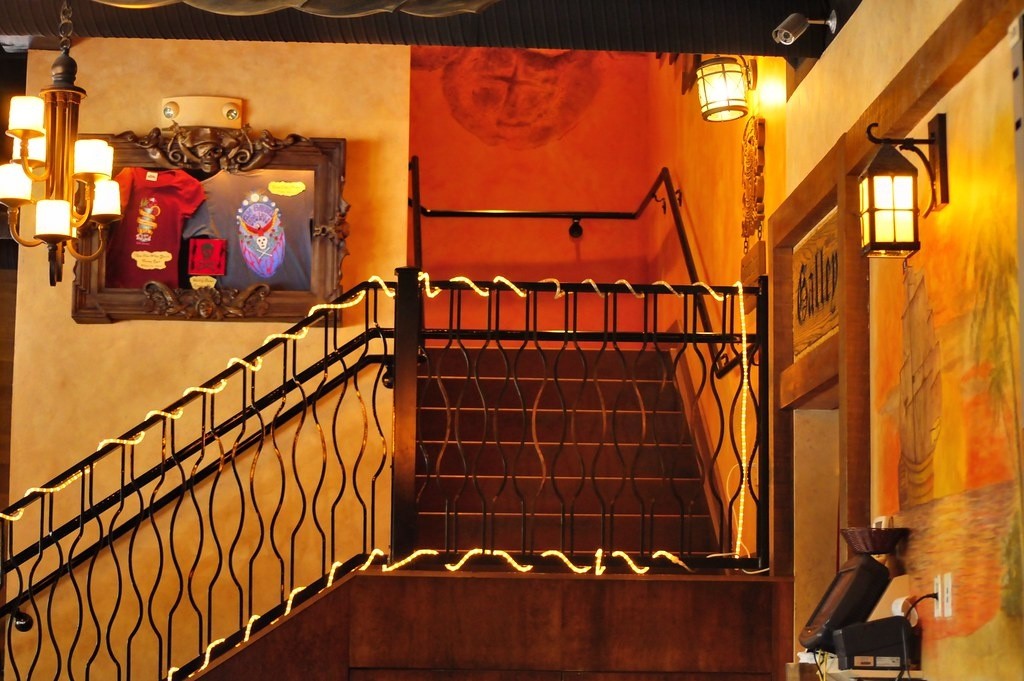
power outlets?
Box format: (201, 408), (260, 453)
(934, 572), (953, 619)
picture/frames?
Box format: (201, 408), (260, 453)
(71, 121), (350, 324)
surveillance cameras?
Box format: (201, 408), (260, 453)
(772, 13), (809, 45)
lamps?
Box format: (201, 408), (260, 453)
(162, 97), (244, 133)
(0, 0), (121, 287)
(856, 111), (948, 259)
(694, 54), (757, 123)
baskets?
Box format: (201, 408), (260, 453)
(840, 527), (910, 554)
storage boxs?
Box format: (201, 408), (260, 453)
(785, 662), (821, 681)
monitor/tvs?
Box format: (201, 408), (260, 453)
(799, 553), (890, 649)
